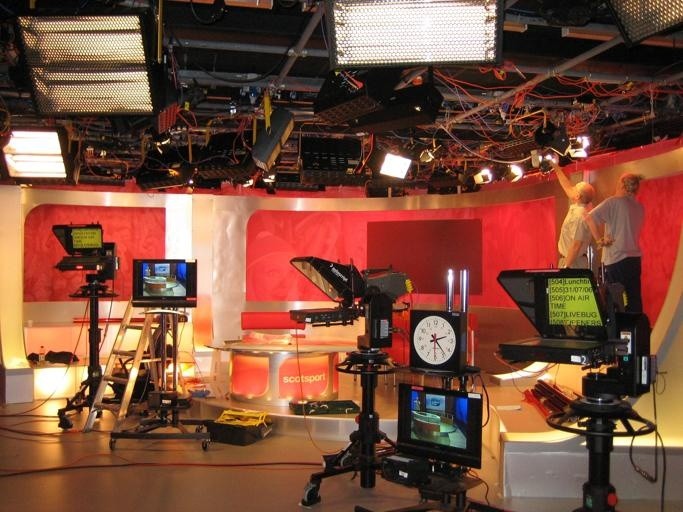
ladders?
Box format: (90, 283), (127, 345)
(82, 295), (159, 433)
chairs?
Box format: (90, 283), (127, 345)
(353, 325), (475, 393)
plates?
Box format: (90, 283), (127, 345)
(191, 389), (210, 398)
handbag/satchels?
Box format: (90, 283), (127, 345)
(290, 398), (361, 416)
(209, 411), (273, 448)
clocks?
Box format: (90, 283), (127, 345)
(408, 309), (464, 374)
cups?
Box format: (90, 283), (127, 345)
(27, 319), (33, 328)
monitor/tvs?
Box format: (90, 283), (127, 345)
(131, 258), (197, 308)
(69, 227), (103, 251)
(542, 277), (606, 335)
(396, 382), (484, 469)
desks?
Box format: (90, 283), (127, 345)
(201, 338), (359, 407)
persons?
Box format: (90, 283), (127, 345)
(145, 266), (150, 276)
(584, 172), (645, 314)
(548, 152), (595, 270)
(413, 396), (421, 411)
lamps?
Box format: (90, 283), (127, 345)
(469, 133), (590, 187)
(240, 90), (296, 173)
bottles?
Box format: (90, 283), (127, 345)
(38, 346), (45, 365)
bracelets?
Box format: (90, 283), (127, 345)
(561, 264), (569, 269)
(595, 236), (604, 245)
